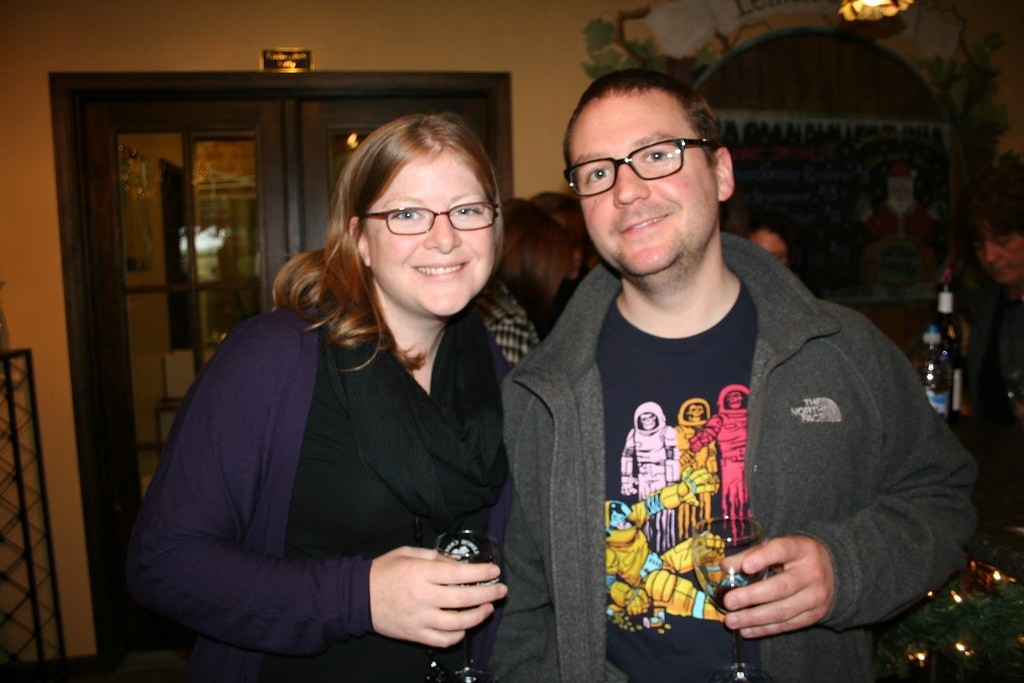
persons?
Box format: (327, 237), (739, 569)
(481, 68), (981, 682)
(123, 112), (516, 683)
(968, 163), (1024, 430)
(477, 189), (600, 372)
(721, 206), (806, 285)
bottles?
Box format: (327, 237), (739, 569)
(918, 318), (949, 425)
(934, 283), (963, 418)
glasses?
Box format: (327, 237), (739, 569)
(362, 201), (499, 235)
(563, 139), (721, 198)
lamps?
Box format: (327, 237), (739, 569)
(836, 0), (915, 23)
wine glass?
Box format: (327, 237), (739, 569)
(433, 530), (504, 683)
(692, 516), (775, 683)
